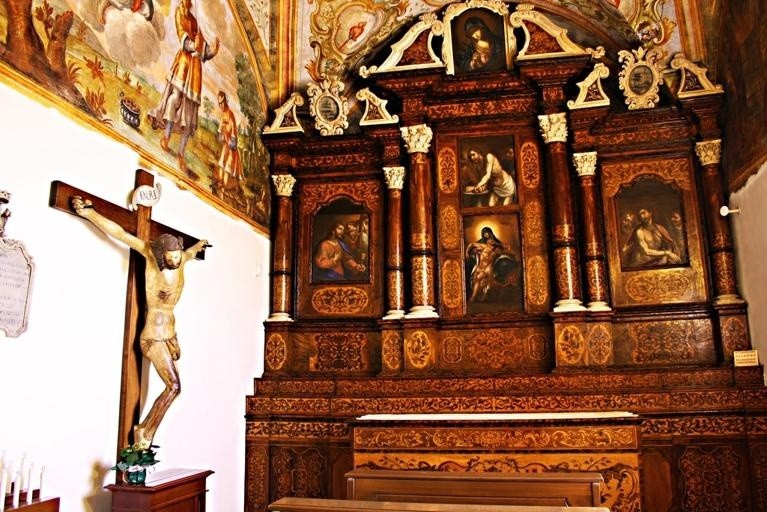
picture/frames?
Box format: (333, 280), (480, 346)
(0, 1), (296, 242)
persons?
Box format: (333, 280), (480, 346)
(620, 206), (685, 267)
(71, 194), (208, 448)
(345, 223), (368, 246)
(460, 144), (516, 206)
(216, 90), (245, 192)
(476, 227), (502, 243)
(159, 1), (221, 173)
(466, 240), (504, 301)
(314, 222), (366, 278)
(460, 17), (505, 70)
